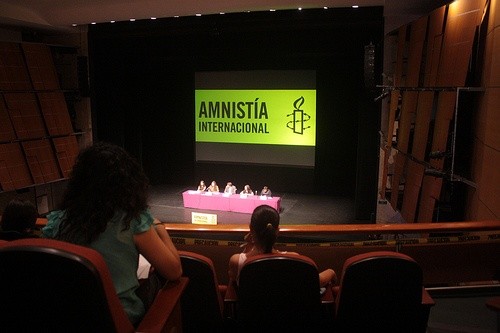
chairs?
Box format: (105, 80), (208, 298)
(149, 250), (221, 333)
(224, 253), (322, 333)
(0, 237), (189, 333)
(323, 251), (434, 333)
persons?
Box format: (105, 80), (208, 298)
(224, 182), (236, 194)
(261, 186), (272, 196)
(198, 180), (206, 190)
(230, 205), (337, 287)
(240, 185), (253, 195)
(40, 145), (183, 326)
(208, 181), (219, 192)
(0, 198), (39, 241)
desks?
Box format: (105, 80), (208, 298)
(182, 190), (282, 214)
